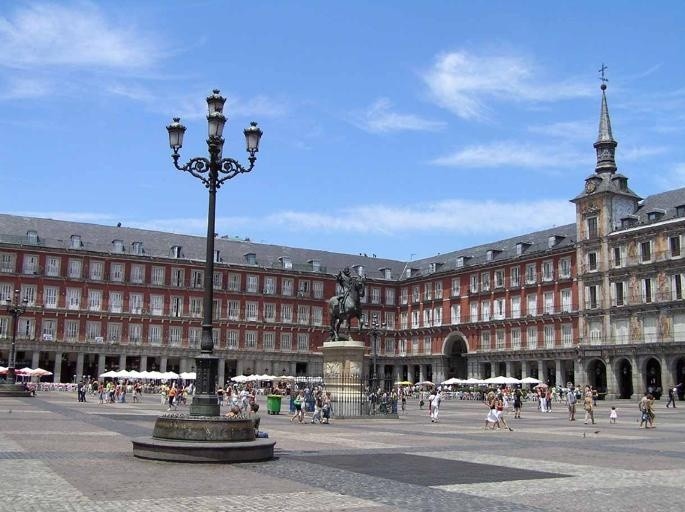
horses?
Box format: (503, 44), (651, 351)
(327, 275), (368, 341)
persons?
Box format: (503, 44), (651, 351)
(336, 265), (363, 317)
(609, 406), (617, 425)
(77, 377), (197, 411)
(24, 382), (37, 395)
(638, 381), (676, 429)
(215, 372), (335, 438)
(368, 384), (599, 431)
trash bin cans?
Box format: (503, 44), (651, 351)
(267, 394), (281, 415)
(36, 383), (42, 391)
(379, 400), (386, 412)
(126, 384), (133, 393)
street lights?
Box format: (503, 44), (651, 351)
(6, 287), (28, 384)
(166, 89), (263, 416)
(364, 315), (387, 379)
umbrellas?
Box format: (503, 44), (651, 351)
(99, 369), (197, 380)
(392, 376), (542, 386)
(0, 365), (54, 384)
